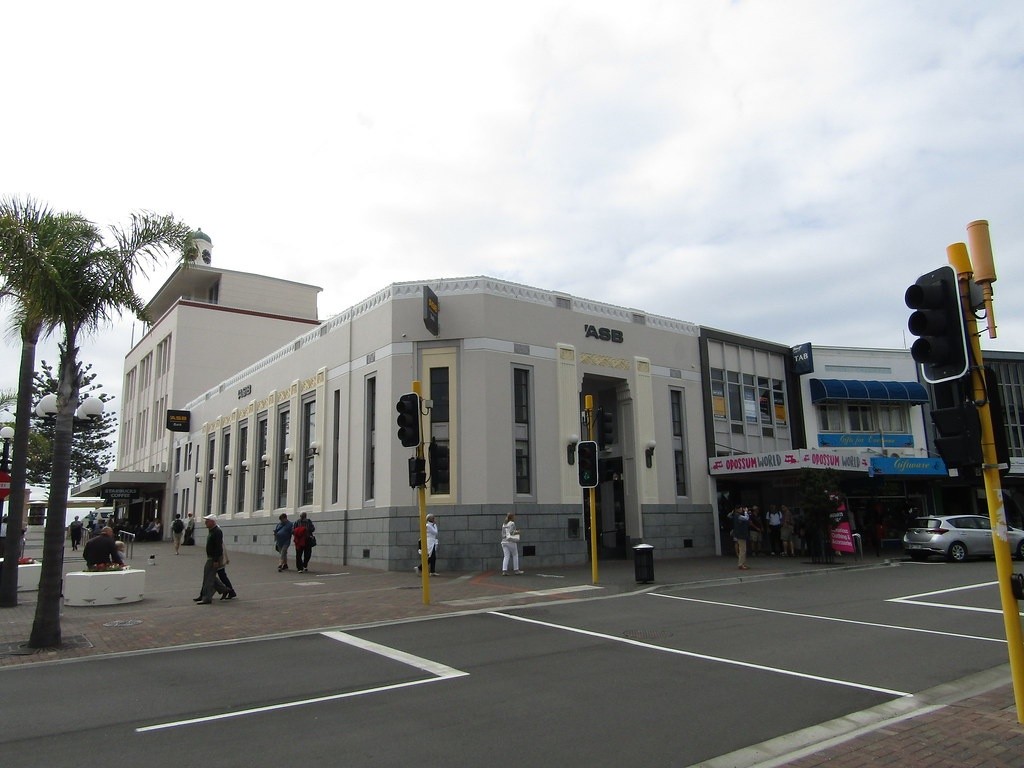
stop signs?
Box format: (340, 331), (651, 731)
(0, 474), (11, 498)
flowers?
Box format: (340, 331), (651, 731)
(83, 563), (124, 572)
(19, 557), (38, 564)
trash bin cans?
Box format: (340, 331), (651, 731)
(632, 544), (655, 584)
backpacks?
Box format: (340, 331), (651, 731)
(173, 520), (183, 533)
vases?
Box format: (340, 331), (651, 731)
(0, 558), (41, 591)
(64, 569), (146, 606)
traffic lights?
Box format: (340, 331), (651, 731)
(408, 456), (426, 489)
(430, 436), (449, 485)
(902, 264), (970, 384)
(395, 392), (423, 449)
(597, 408), (617, 451)
(935, 403), (984, 466)
(576, 440), (599, 488)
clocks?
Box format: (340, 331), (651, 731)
(187, 247), (198, 261)
(202, 249), (211, 264)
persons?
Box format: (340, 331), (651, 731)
(733, 505), (751, 569)
(83, 526), (124, 569)
(181, 513), (193, 545)
(71, 516), (81, 551)
(86, 515), (160, 542)
(293, 512), (315, 573)
(413, 513), (440, 576)
(115, 540), (124, 563)
(727, 504), (795, 558)
(170, 514), (184, 554)
(275, 513), (293, 571)
(192, 514), (237, 604)
(501, 513), (523, 575)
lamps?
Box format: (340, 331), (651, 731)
(567, 434), (579, 465)
(284, 448), (292, 460)
(310, 442), (319, 456)
(196, 473), (201, 482)
(262, 455), (269, 466)
(645, 440), (656, 468)
(210, 469), (215, 479)
(242, 461), (249, 472)
(225, 465), (231, 475)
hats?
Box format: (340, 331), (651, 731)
(735, 504), (741, 508)
(204, 514), (217, 521)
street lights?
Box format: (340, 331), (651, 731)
(0, 427), (15, 536)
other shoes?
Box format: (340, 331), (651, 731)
(781, 554), (788, 557)
(193, 596), (202, 601)
(739, 565), (748, 570)
(196, 600), (211, 604)
(283, 564), (287, 569)
(304, 567), (308, 571)
(298, 569), (303, 573)
(414, 567), (421, 576)
(220, 591), (228, 600)
(225, 590), (236, 599)
(515, 570), (524, 575)
(430, 573), (440, 576)
(503, 571), (510, 576)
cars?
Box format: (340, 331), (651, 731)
(902, 513), (1024, 561)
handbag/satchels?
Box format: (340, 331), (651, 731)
(309, 536), (316, 547)
(505, 522), (520, 542)
